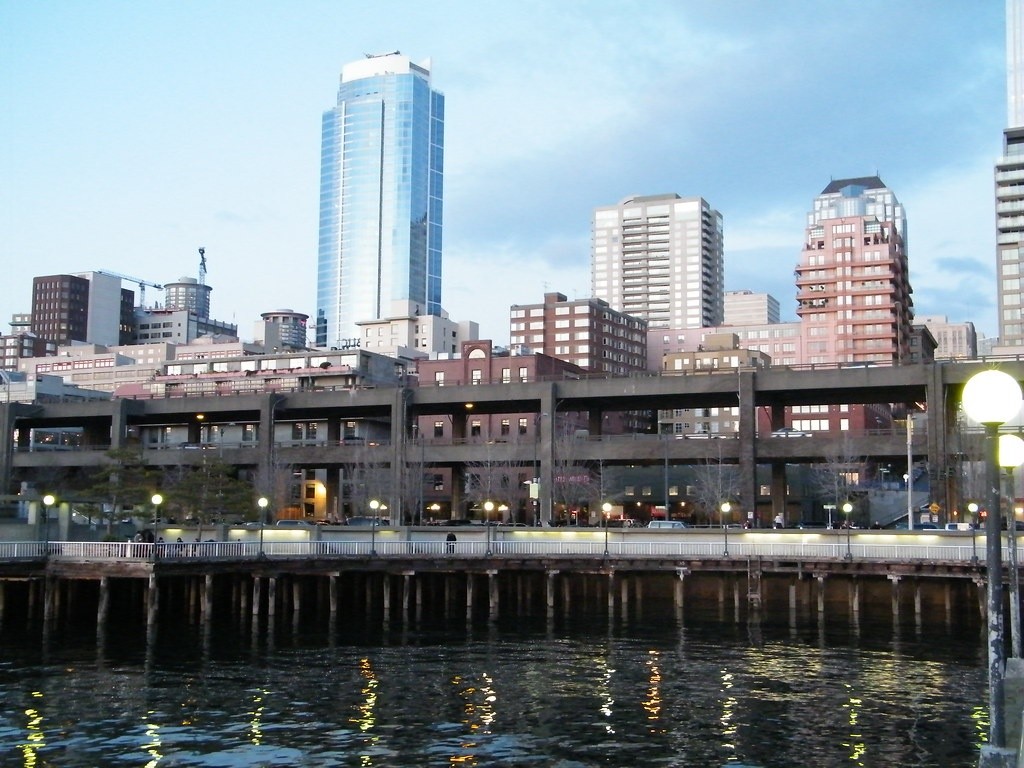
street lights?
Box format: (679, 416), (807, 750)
(843, 503), (852, 552)
(995, 433), (1024, 661)
(602, 503), (612, 555)
(657, 420), (670, 521)
(903, 474), (910, 491)
(967, 501), (978, 557)
(412, 424), (425, 530)
(43, 494), (55, 560)
(720, 503), (731, 557)
(959, 370), (1024, 750)
(368, 500), (380, 556)
(532, 413), (548, 528)
(485, 502), (494, 557)
(152, 494), (164, 560)
(256, 498), (269, 557)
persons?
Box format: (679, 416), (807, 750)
(158, 537), (165, 546)
(773, 514), (783, 529)
(134, 531), (143, 542)
(177, 537), (185, 551)
(446, 532), (457, 553)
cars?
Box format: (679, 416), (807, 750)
(675, 429), (726, 440)
(791, 521), (828, 529)
(886, 518), (1024, 532)
(554, 518), (644, 528)
(770, 427), (808, 437)
(407, 519), (529, 528)
(237, 516), (389, 527)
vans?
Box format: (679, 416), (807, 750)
(646, 520), (690, 529)
